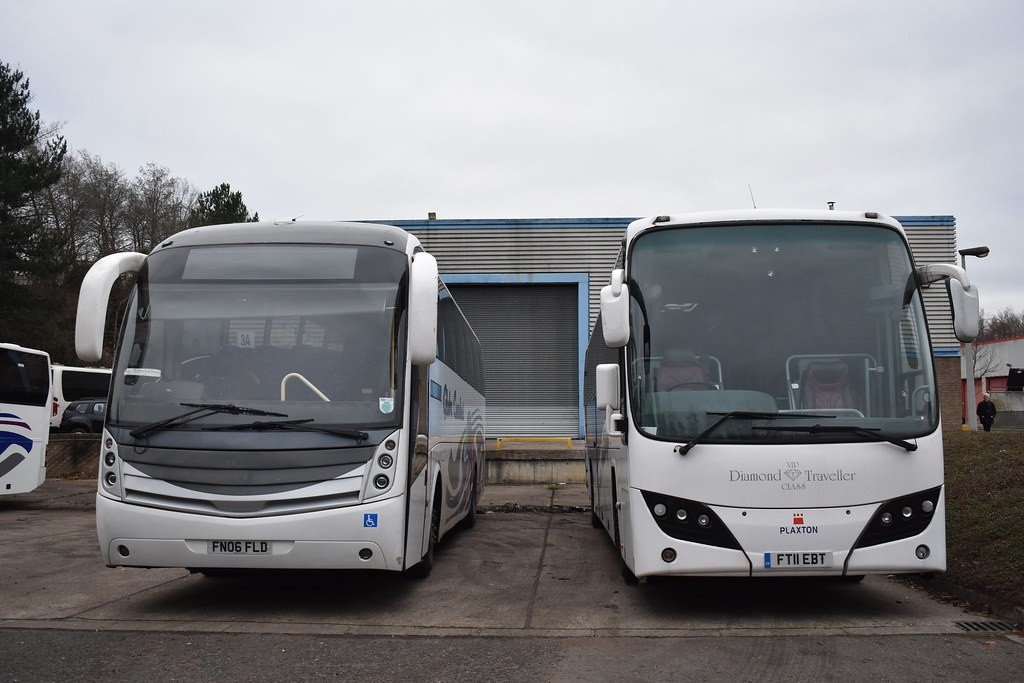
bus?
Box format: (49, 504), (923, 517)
(75, 216), (488, 583)
(0, 341), (162, 496)
(581, 208), (981, 588)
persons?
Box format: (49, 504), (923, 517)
(977, 393), (996, 433)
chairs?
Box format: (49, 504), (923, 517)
(655, 347), (705, 392)
(213, 342), (390, 403)
(801, 361), (856, 411)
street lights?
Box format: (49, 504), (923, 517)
(958, 244), (990, 431)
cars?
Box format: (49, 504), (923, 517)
(59, 398), (107, 434)
